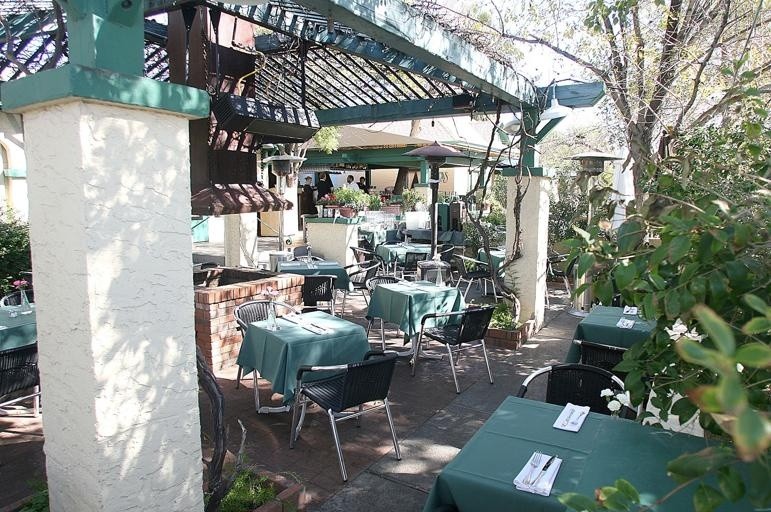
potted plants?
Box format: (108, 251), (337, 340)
(333, 186), (371, 218)
(400, 190), (427, 211)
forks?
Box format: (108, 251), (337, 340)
(570, 411), (586, 426)
(522, 449), (543, 485)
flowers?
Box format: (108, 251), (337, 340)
(379, 192), (392, 207)
(314, 192), (338, 203)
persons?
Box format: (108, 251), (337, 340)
(345, 175), (360, 190)
(304, 176), (317, 213)
(317, 171), (331, 200)
(357, 177), (369, 194)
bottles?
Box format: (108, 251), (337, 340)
(435, 267), (443, 287)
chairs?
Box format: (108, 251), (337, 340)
(0, 287), (35, 308)
(610, 293), (631, 306)
(514, 362), (629, 419)
(0, 341), (41, 409)
(571, 338), (649, 403)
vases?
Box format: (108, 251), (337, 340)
(325, 204), (338, 211)
(381, 205), (399, 218)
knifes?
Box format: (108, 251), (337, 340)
(311, 323), (328, 331)
(532, 454), (560, 488)
(561, 408), (575, 428)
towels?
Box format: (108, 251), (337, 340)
(552, 402), (591, 432)
(616, 318), (636, 329)
(512, 452), (563, 497)
(623, 305), (637, 315)
